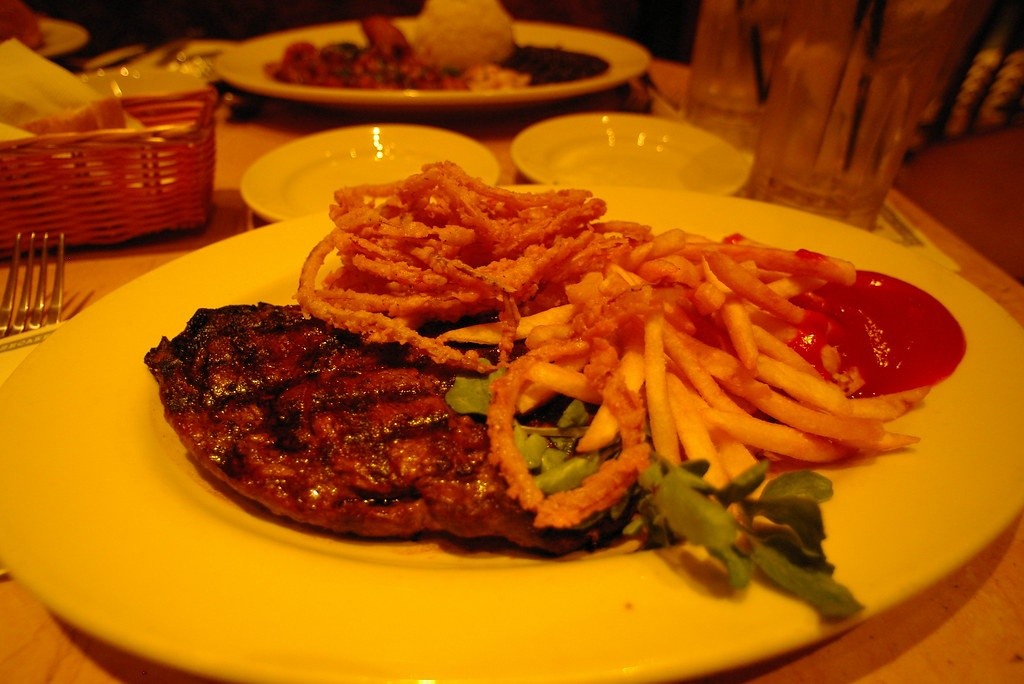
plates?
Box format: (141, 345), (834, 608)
(242, 125), (502, 223)
(510, 111), (749, 201)
(0, 20), (87, 59)
(80, 69), (207, 100)
(0, 184), (1024, 684)
(212, 17), (655, 116)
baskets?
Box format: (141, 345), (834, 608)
(0, 86), (216, 257)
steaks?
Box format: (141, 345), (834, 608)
(146, 305), (635, 556)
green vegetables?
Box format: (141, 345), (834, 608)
(445, 354), (869, 620)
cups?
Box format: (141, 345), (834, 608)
(680, 0), (776, 149)
(753, 0), (964, 229)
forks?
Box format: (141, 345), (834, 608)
(0, 234), (64, 336)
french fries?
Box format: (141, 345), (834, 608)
(419, 228), (932, 482)
